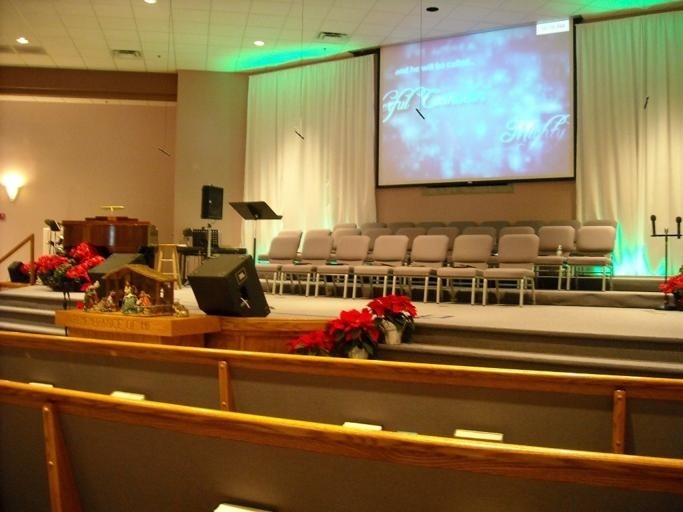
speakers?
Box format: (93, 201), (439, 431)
(201, 185), (223, 220)
(187, 254), (270, 317)
(7, 261), (29, 283)
(87, 253), (147, 297)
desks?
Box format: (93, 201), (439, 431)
(178, 246), (246, 281)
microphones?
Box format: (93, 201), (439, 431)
(676, 217), (681, 238)
(651, 215), (656, 235)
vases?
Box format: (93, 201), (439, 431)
(380, 319), (406, 345)
(348, 348), (369, 359)
(675, 295), (683, 312)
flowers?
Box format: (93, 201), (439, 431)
(658, 274), (683, 294)
(366, 294), (416, 327)
(326, 310), (381, 355)
(286, 330), (335, 355)
(19, 242), (103, 286)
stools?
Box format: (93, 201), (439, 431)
(158, 243), (181, 291)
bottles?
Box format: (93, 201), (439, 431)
(556, 244), (562, 256)
